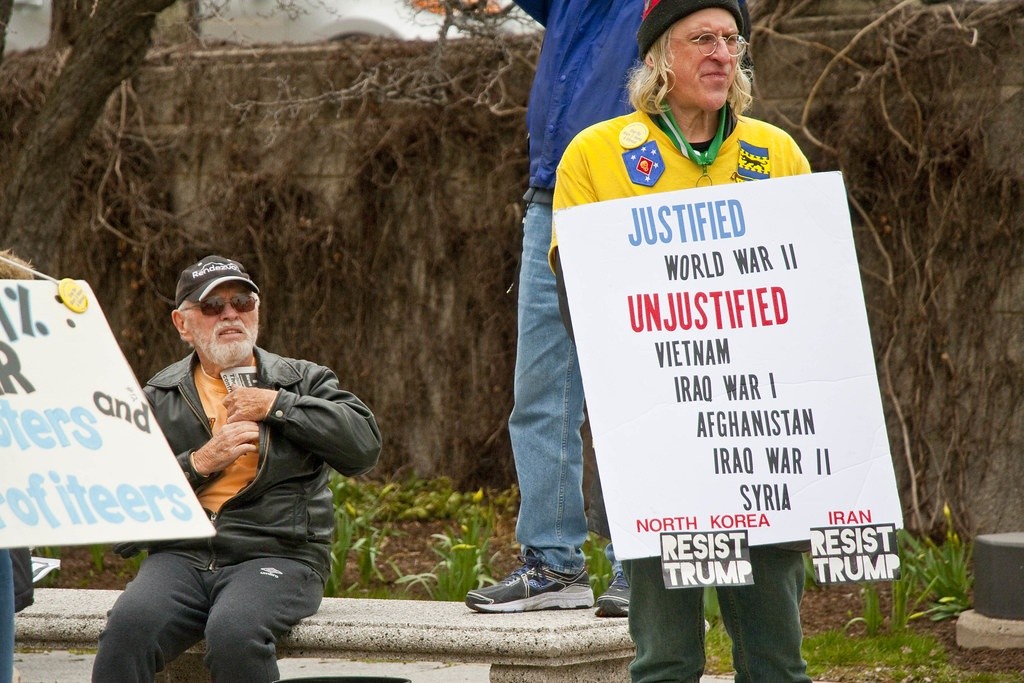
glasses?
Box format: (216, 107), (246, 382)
(671, 33), (750, 57)
(180, 293), (257, 315)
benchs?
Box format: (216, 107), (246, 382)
(14, 587), (712, 683)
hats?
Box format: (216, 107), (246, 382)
(175, 255), (260, 309)
(637, 0), (752, 67)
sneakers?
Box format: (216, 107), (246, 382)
(466, 549), (595, 612)
(595, 572), (631, 617)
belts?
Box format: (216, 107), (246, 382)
(523, 187), (553, 204)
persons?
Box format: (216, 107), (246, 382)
(92, 256), (381, 683)
(549, 0), (813, 682)
(0, 249), (34, 683)
(466, 0), (645, 615)
